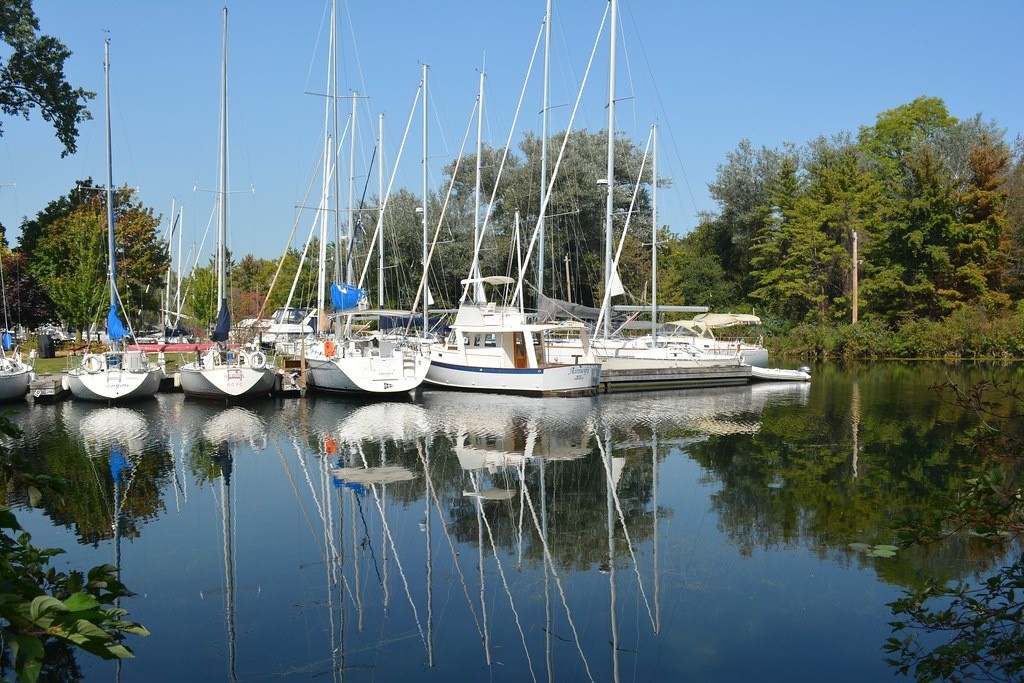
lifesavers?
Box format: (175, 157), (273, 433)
(249, 351), (267, 370)
(82, 354), (103, 374)
(324, 342), (335, 357)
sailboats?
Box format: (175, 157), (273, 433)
(58, 28), (165, 405)
(286, 394), (672, 683)
(164, 4), (286, 403)
(77, 405), (148, 683)
(278, 0), (813, 398)
(0, 258), (41, 403)
(200, 403), (265, 683)
